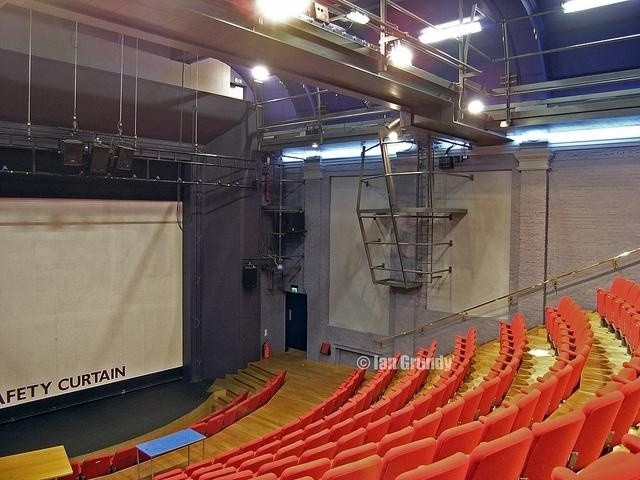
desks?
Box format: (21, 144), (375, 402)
(0, 443), (75, 479)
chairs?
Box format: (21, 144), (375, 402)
(203, 369), (353, 425)
(353, 271), (639, 480)
(59, 425), (353, 480)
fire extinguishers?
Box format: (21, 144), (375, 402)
(263, 339), (271, 359)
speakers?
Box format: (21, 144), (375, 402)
(244, 264), (257, 289)
